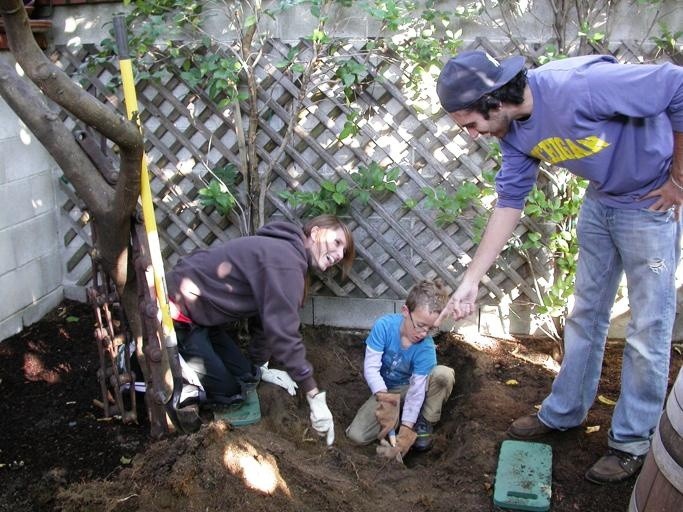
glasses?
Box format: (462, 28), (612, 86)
(408, 311), (439, 337)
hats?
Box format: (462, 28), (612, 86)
(435, 50), (525, 113)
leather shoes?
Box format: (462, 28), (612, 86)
(505, 412), (558, 441)
(584, 447), (646, 486)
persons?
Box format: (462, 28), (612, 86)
(94, 211), (355, 447)
(431, 49), (682, 485)
(343, 277), (456, 462)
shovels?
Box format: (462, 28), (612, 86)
(114, 12), (202, 434)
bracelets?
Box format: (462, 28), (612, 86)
(668, 172), (682, 192)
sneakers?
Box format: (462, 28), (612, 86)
(412, 415), (433, 451)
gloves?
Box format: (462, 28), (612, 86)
(260, 360), (298, 397)
(375, 425), (417, 465)
(305, 391), (334, 446)
(374, 393), (400, 441)
